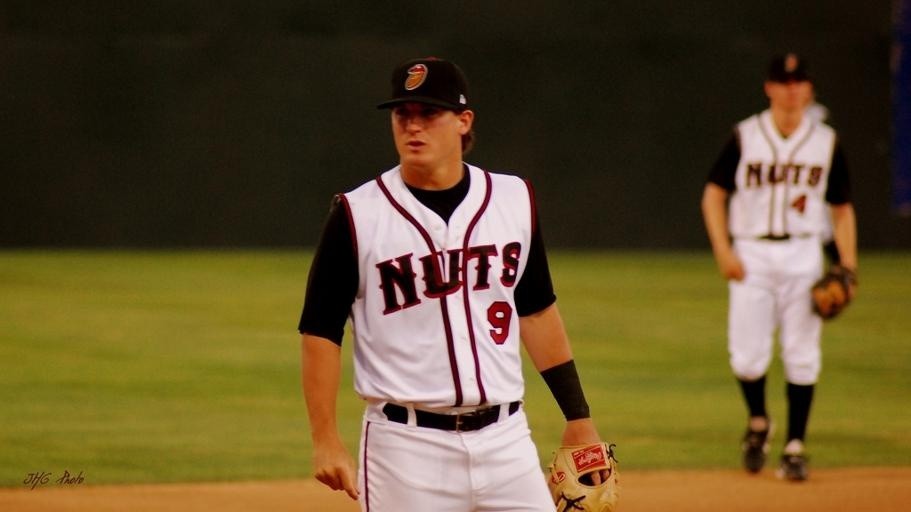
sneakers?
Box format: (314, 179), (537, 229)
(745, 415), (772, 472)
(779, 453), (807, 481)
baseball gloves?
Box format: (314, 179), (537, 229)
(812, 266), (857, 319)
(548, 443), (618, 511)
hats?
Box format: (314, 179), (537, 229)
(768, 52), (808, 82)
(378, 58), (470, 111)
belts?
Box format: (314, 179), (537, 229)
(764, 235), (791, 240)
(384, 403), (519, 430)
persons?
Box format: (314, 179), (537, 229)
(699, 51), (862, 483)
(297, 57), (623, 512)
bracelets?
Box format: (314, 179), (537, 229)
(539, 358), (592, 422)
